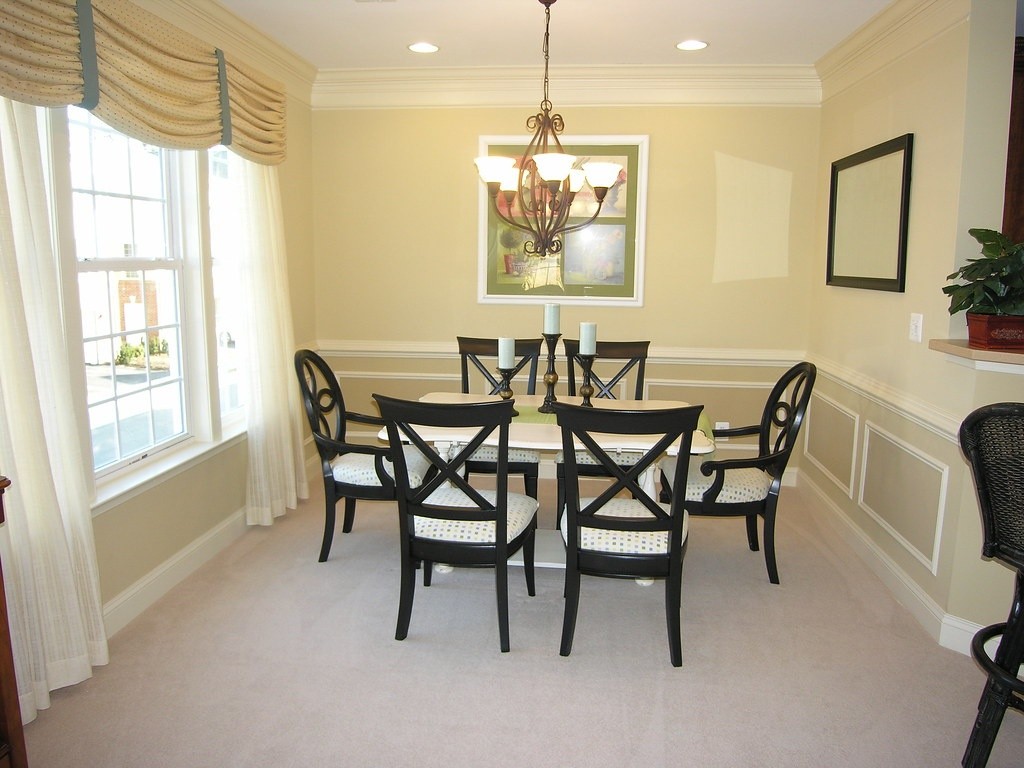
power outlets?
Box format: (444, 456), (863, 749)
(714, 422), (730, 440)
(908, 312), (923, 343)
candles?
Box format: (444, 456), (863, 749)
(543, 302), (560, 334)
(580, 320), (597, 355)
(498, 337), (515, 369)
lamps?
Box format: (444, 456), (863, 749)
(473, 0), (623, 257)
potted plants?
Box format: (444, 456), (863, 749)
(941, 228), (1024, 349)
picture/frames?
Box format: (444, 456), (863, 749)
(824, 133), (914, 292)
(477, 133), (651, 307)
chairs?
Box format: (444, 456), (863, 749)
(956, 400), (1024, 768)
(455, 336), (545, 527)
(557, 338), (658, 530)
(372, 392), (539, 653)
(549, 403), (706, 668)
(659, 361), (818, 586)
(293, 349), (469, 562)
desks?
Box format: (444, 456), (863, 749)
(378, 391), (716, 586)
